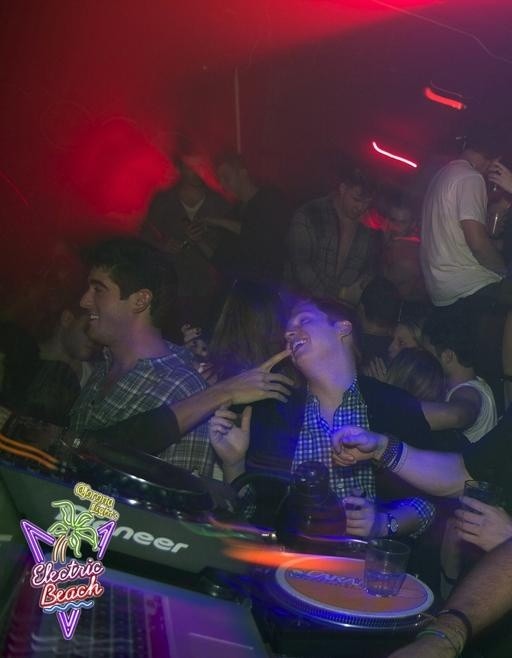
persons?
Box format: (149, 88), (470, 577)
(0, 133), (512, 658)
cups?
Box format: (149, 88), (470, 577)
(362, 538), (413, 597)
(462, 478), (504, 517)
